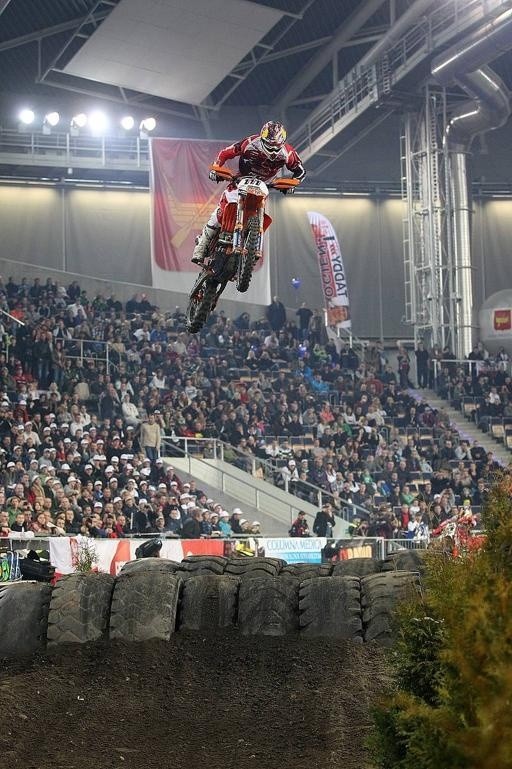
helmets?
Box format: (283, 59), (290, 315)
(260, 120), (286, 161)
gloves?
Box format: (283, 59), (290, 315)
(209, 169), (220, 184)
(287, 188), (295, 194)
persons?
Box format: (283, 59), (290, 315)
(190, 119), (307, 265)
(0, 275), (512, 549)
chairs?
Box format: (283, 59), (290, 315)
(229, 368), (512, 517)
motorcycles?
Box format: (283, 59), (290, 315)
(185, 165), (300, 334)
(432, 510), (489, 560)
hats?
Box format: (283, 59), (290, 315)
(299, 511), (305, 515)
(434, 495), (441, 499)
(289, 460), (296, 465)
(0, 400), (260, 526)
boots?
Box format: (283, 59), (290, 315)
(191, 224), (218, 263)
